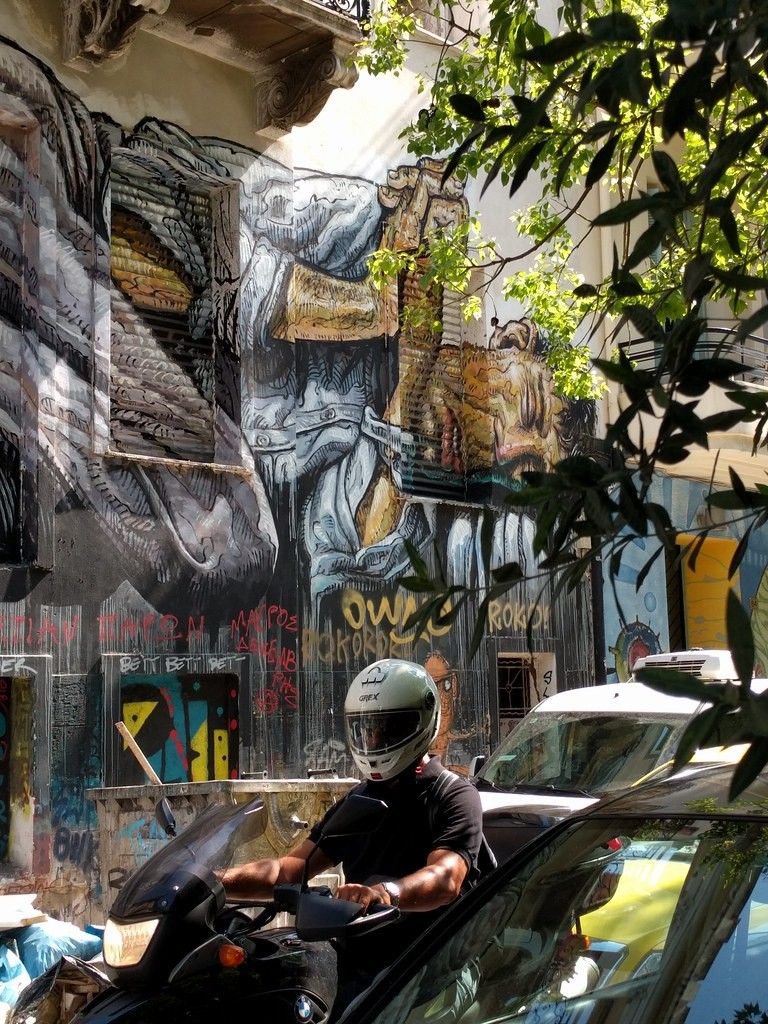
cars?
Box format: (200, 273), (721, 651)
(536, 749), (768, 997)
(333, 765), (768, 1023)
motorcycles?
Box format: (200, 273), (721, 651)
(68, 793), (625, 1023)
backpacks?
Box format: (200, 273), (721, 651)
(422, 769), (498, 879)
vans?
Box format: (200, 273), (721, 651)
(471, 648), (768, 819)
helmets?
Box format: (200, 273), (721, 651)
(343, 658), (441, 782)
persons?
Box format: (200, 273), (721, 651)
(212, 658), (482, 1024)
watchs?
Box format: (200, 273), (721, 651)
(381, 882), (400, 907)
